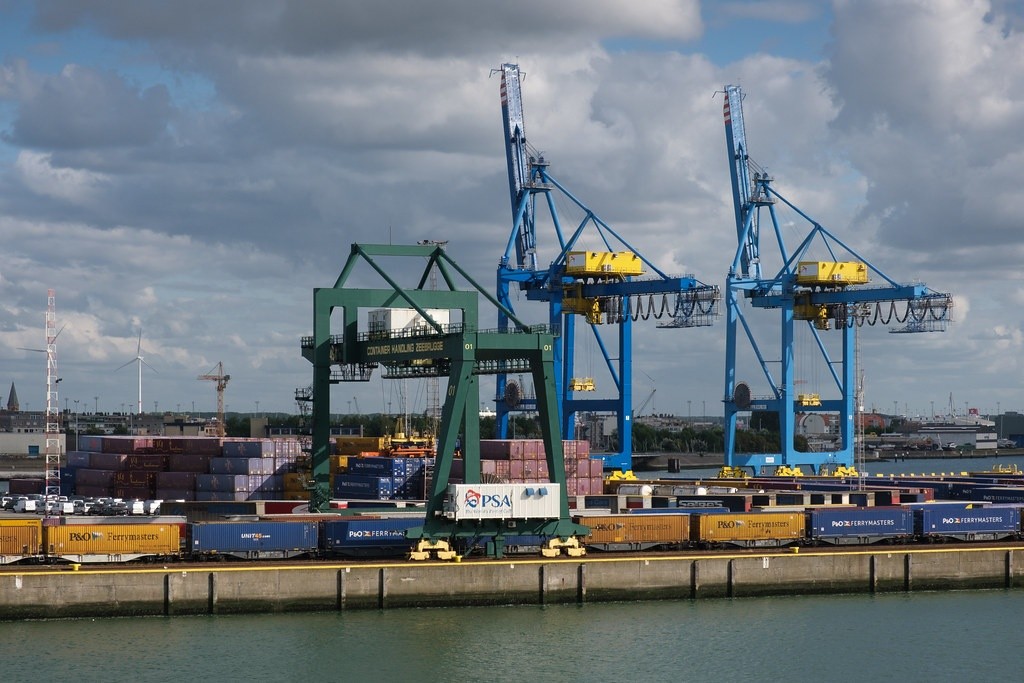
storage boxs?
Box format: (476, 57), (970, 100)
(0, 430), (1024, 552)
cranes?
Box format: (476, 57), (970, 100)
(195, 361), (232, 437)
(490, 63), (722, 473)
(710, 82), (955, 476)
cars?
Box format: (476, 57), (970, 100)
(0, 491), (186, 516)
